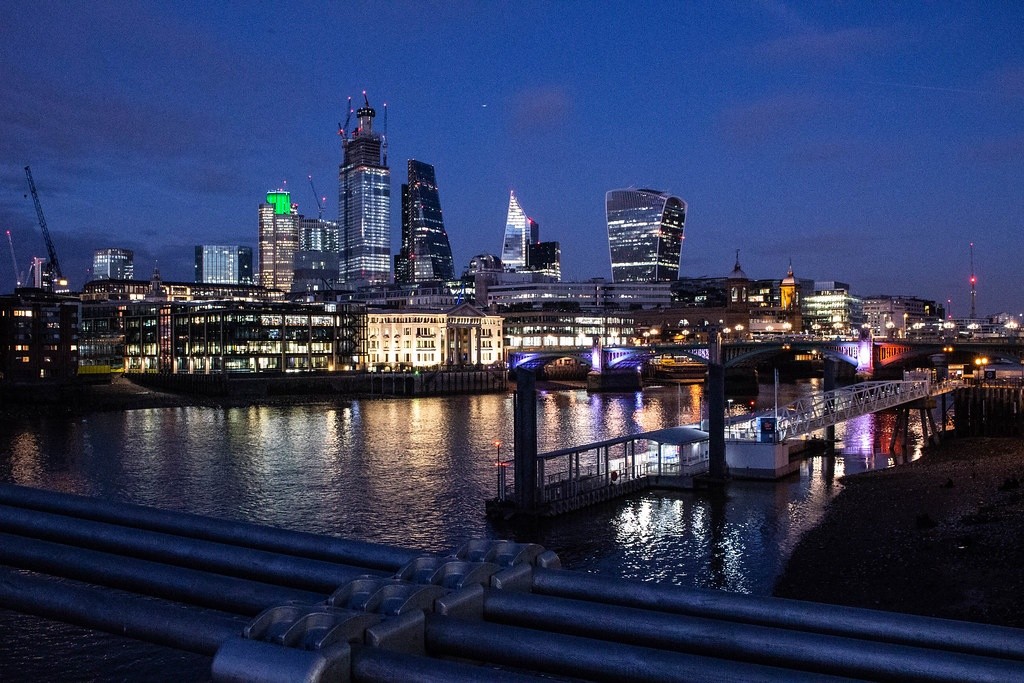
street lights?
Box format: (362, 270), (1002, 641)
(766, 326), (774, 334)
(728, 399), (734, 439)
(903, 312), (908, 337)
(975, 358), (987, 384)
(943, 347), (952, 385)
(651, 329), (660, 364)
(682, 329), (689, 343)
(783, 322), (790, 342)
(724, 327), (731, 342)
(642, 332), (650, 346)
(735, 323), (744, 341)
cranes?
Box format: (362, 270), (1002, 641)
(380, 102), (392, 169)
(5, 165), (68, 289)
(309, 174), (326, 219)
(337, 96), (354, 279)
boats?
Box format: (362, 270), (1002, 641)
(652, 362), (706, 385)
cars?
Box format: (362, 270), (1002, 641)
(970, 324), (1017, 339)
